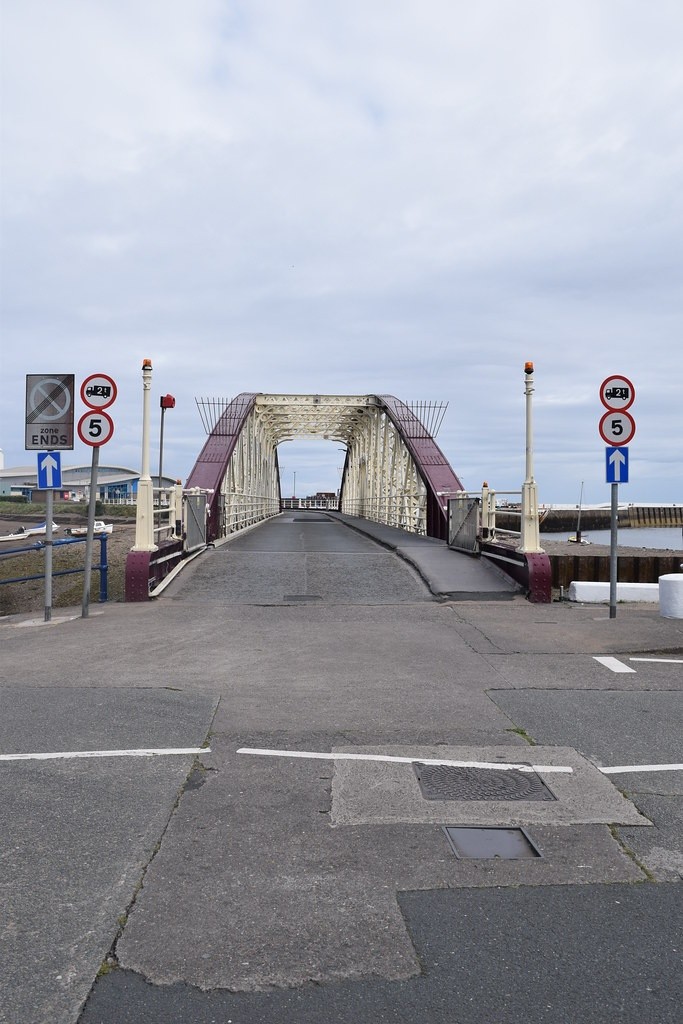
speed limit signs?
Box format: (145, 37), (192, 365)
(599, 409), (636, 446)
(77, 409), (115, 447)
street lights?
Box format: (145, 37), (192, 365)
(157, 394), (176, 543)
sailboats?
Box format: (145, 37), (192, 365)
(567, 481), (591, 544)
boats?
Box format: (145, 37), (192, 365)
(63, 520), (113, 536)
(26, 519), (61, 535)
(0, 525), (31, 541)
